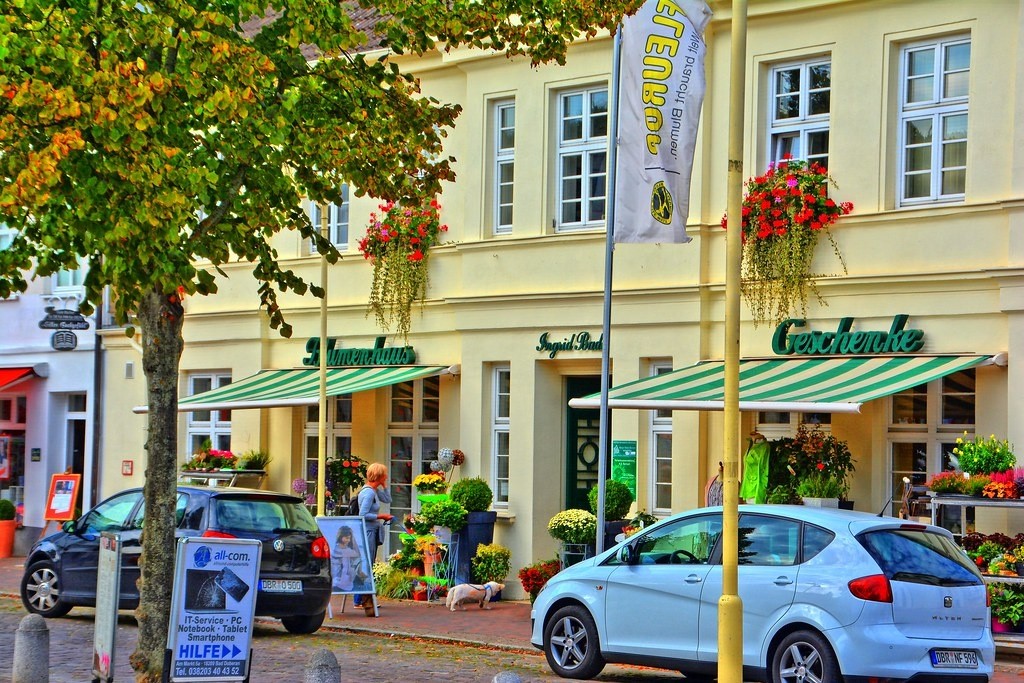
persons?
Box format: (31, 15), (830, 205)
(0, 439), (7, 465)
(329, 526), (370, 592)
(707, 466), (746, 560)
(354, 462), (395, 614)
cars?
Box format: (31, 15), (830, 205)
(18, 486), (335, 636)
(529, 501), (998, 683)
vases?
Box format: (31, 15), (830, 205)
(565, 540), (591, 569)
(406, 527), (415, 534)
(484, 572), (494, 584)
(182, 467), (266, 476)
(434, 525), (451, 542)
(411, 565), (424, 576)
(838, 501), (854, 510)
(411, 592), (427, 601)
(422, 489), (446, 494)
(1014, 562), (1024, 576)
(438, 596), (447, 601)
(991, 616), (1012, 633)
(624, 539), (652, 566)
(424, 555), (440, 563)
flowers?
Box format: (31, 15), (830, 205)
(924, 431), (1024, 500)
(621, 509), (659, 537)
(354, 197), (464, 354)
(547, 508), (597, 553)
(771, 419), (859, 501)
(516, 552), (569, 603)
(959, 532), (1024, 573)
(721, 154), (853, 349)
(470, 543), (513, 585)
(987, 581), (1024, 626)
(373, 474), (468, 601)
(325, 450), (369, 503)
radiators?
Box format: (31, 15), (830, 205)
(180, 440), (273, 472)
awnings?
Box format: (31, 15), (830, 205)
(569, 354), (1009, 415)
(0, 363), (50, 393)
(134, 364), (461, 414)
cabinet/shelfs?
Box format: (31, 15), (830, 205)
(930, 498), (1024, 657)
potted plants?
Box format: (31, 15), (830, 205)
(449, 475), (497, 586)
(0, 499), (16, 559)
(792, 468), (844, 508)
(588, 478), (633, 561)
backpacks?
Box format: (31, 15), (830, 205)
(343, 485), (376, 516)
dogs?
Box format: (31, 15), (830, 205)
(446, 581), (505, 611)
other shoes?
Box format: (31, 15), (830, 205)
(355, 604), (366, 610)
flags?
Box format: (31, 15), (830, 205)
(614, 0), (712, 245)
